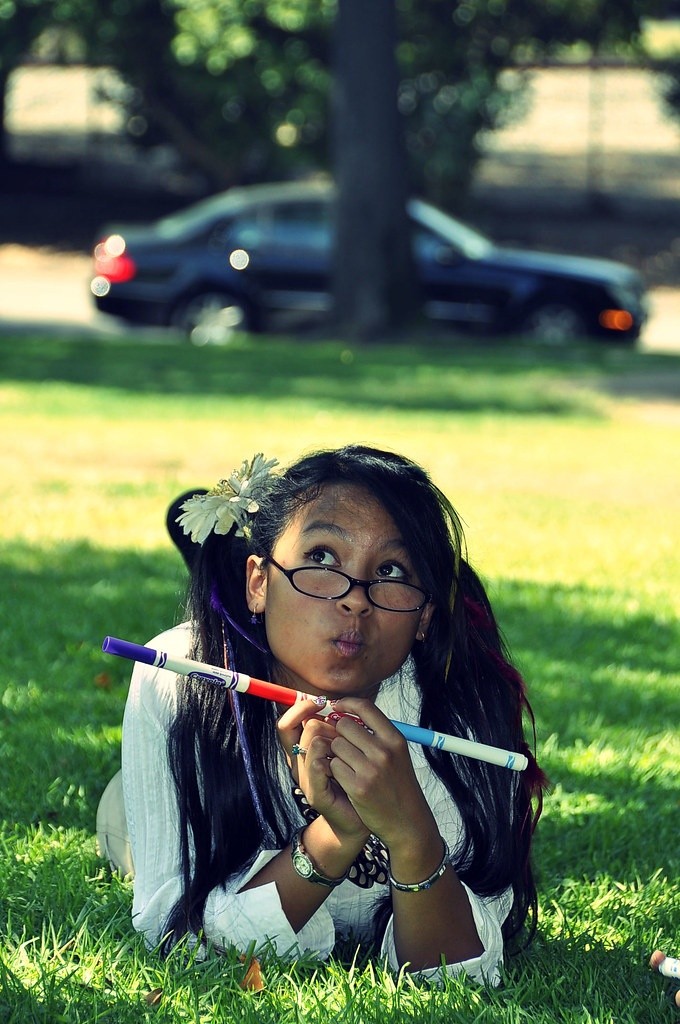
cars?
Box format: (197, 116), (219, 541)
(87, 178), (651, 354)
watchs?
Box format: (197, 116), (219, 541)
(291, 824), (349, 887)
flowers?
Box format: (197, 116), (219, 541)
(175, 452), (280, 547)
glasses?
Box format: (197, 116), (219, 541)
(261, 554), (434, 612)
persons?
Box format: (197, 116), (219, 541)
(100, 445), (545, 993)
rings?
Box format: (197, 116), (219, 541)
(292, 743), (309, 756)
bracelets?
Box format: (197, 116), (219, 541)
(385, 835), (451, 892)
